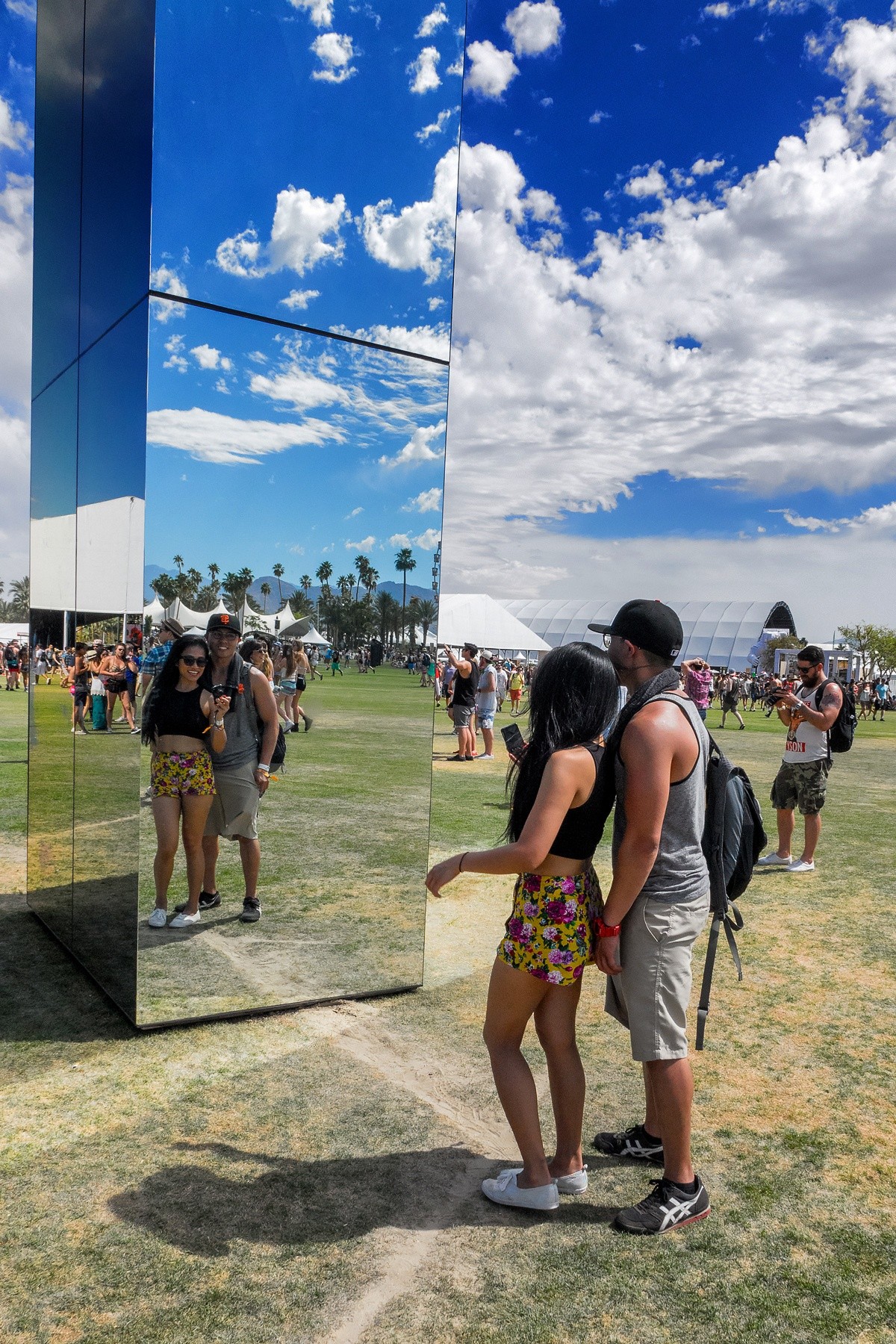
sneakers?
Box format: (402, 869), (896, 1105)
(482, 1177), (561, 1211)
(447, 754), (466, 762)
(593, 1124), (665, 1164)
(465, 755), (474, 761)
(783, 858), (814, 872)
(613, 1175), (711, 1235)
(499, 1164), (590, 1196)
(477, 752), (494, 759)
(757, 852), (792, 865)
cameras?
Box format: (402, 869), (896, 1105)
(211, 684), (225, 704)
(501, 723), (525, 756)
(766, 687), (788, 706)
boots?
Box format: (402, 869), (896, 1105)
(290, 722), (299, 732)
(283, 720), (295, 734)
(302, 715), (313, 731)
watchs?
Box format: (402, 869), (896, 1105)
(592, 913), (623, 937)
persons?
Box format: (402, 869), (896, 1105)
(0, 554), (896, 789)
(581, 598), (729, 1239)
(425, 638), (625, 1212)
(175, 612), (282, 921)
(137, 632), (232, 930)
(753, 646), (844, 872)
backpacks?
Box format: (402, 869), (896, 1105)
(421, 653), (431, 666)
(240, 661), (288, 774)
(794, 679), (858, 753)
(703, 724), (768, 922)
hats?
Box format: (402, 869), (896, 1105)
(92, 639), (105, 646)
(206, 613), (241, 635)
(273, 641), (283, 647)
(589, 599), (684, 660)
(161, 616), (185, 637)
(481, 650), (493, 661)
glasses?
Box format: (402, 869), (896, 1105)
(117, 648), (125, 651)
(602, 634), (641, 652)
(208, 630), (240, 641)
(158, 628), (169, 633)
(462, 648), (472, 652)
(480, 656), (486, 660)
(796, 663), (820, 673)
(252, 649), (268, 654)
(179, 655), (210, 667)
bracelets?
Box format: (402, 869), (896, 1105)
(459, 850), (471, 874)
